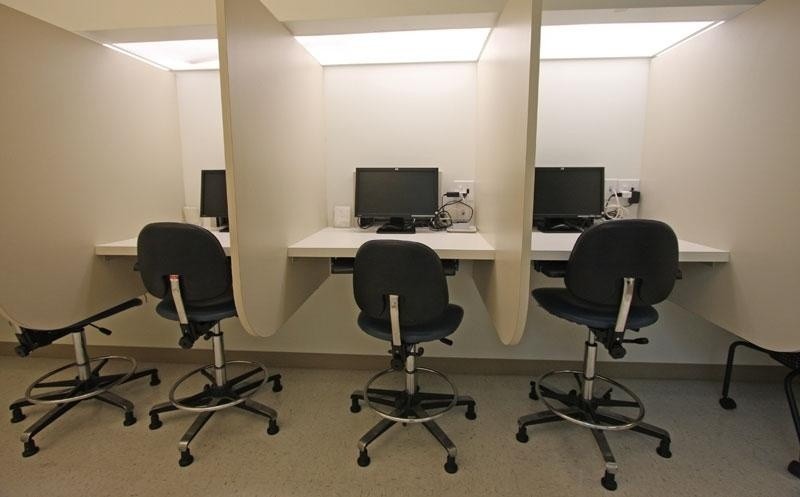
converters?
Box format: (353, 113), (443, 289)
(447, 191), (459, 197)
(619, 191), (633, 199)
(629, 192), (640, 203)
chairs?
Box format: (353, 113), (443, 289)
(515, 218), (678, 489)
(349, 239), (477, 475)
(137, 219), (282, 468)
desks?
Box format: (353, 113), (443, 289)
(286, 227), (496, 265)
(93, 229), (232, 257)
(530, 226), (731, 263)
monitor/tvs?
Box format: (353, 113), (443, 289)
(533, 166), (606, 233)
(353, 167), (438, 234)
(199, 169), (229, 232)
